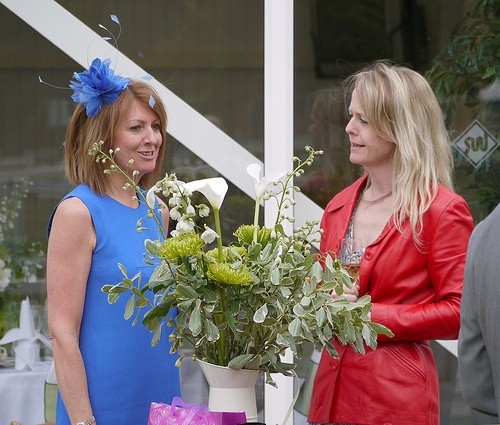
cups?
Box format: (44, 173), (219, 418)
(341, 238), (364, 286)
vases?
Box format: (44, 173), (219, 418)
(195, 359), (260, 422)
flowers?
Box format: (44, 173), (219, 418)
(37, 15), (131, 118)
(88, 142), (395, 386)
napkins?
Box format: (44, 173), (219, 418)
(0, 298), (54, 369)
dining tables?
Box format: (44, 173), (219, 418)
(0, 357), (53, 425)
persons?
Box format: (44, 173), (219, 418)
(298, 88), (365, 207)
(458, 202), (500, 425)
(44, 79), (184, 425)
(304, 62), (475, 425)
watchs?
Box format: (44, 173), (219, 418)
(72, 416), (96, 425)
